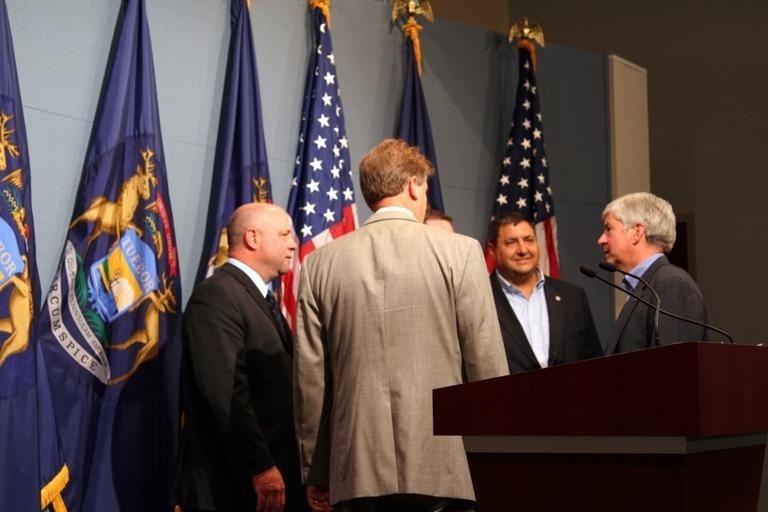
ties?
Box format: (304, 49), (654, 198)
(267, 291), (286, 336)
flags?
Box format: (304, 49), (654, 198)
(481, 39), (562, 278)
(31, 1), (186, 512)
(278, 0), (361, 340)
(384, 17), (447, 216)
(194, 0), (283, 310)
(0, 0), (74, 512)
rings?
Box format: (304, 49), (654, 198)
(312, 495), (319, 503)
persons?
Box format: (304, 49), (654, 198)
(423, 209), (455, 234)
(173, 202), (315, 512)
(595, 191), (708, 357)
(290, 136), (510, 512)
(488, 208), (607, 375)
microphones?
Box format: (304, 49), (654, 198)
(599, 262), (662, 347)
(579, 265), (735, 343)
